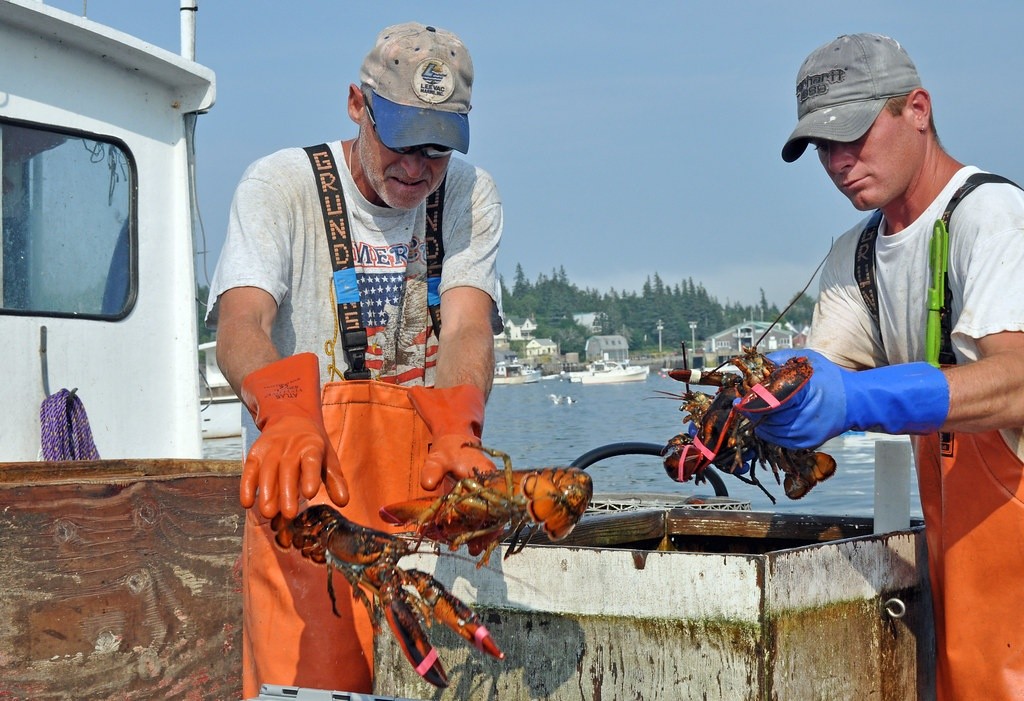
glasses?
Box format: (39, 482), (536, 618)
(361, 89), (456, 159)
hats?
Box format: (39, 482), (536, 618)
(359, 20), (474, 154)
(781, 33), (922, 164)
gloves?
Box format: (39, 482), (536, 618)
(240, 353), (349, 521)
(732, 347), (950, 450)
(688, 421), (757, 474)
(406, 383), (498, 491)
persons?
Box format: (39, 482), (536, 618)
(688, 34), (1024, 701)
(206, 22), (504, 701)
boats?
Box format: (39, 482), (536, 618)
(491, 355), (543, 385)
(660, 362), (742, 374)
(559, 350), (652, 385)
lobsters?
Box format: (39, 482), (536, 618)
(642, 237), (837, 501)
(270, 442), (593, 688)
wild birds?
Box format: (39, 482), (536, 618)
(550, 394), (578, 405)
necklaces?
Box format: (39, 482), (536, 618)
(349, 137), (405, 232)
